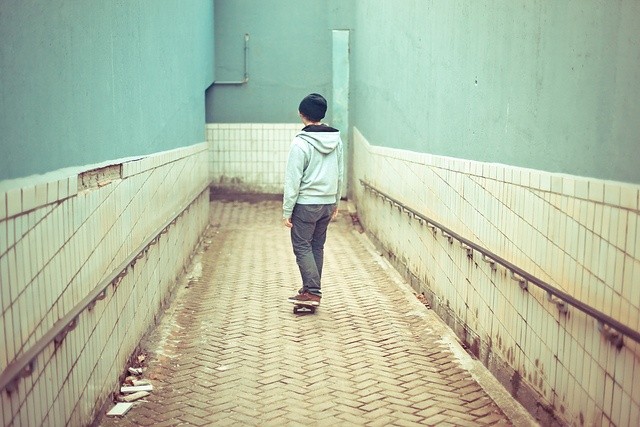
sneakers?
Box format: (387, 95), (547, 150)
(298, 288), (305, 293)
(287, 293), (322, 306)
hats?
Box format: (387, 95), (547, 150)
(299, 93), (327, 119)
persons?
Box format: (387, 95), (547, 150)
(282, 93), (344, 307)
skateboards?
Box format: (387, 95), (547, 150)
(294, 305), (315, 313)
